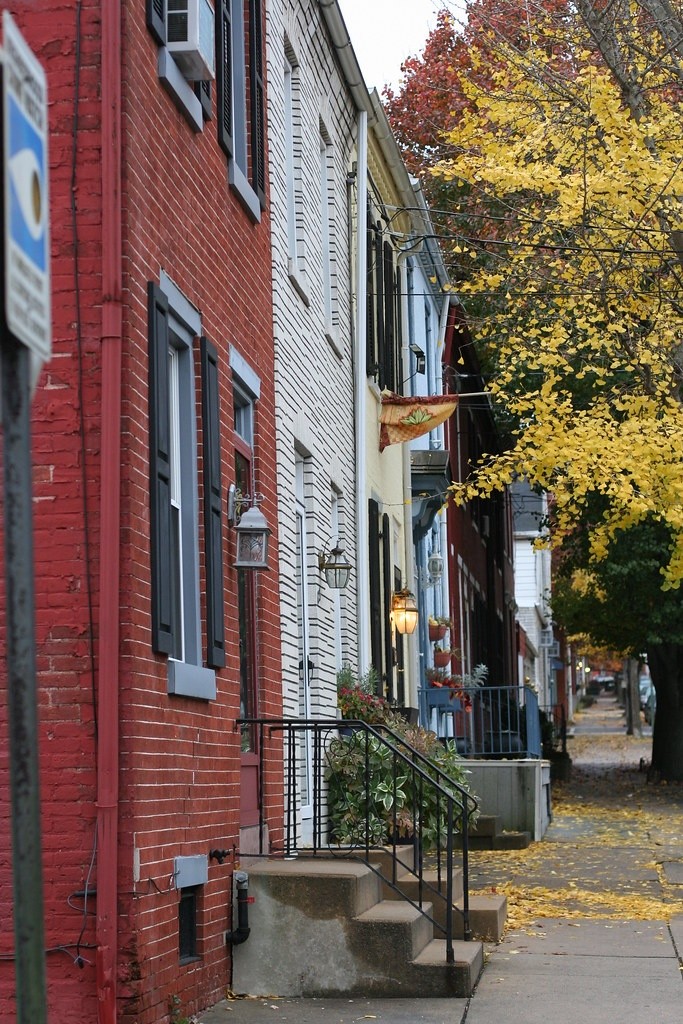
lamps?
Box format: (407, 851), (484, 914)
(319, 535), (352, 589)
(419, 550), (444, 592)
(228, 484), (274, 571)
(392, 585), (418, 634)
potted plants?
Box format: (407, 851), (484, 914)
(429, 617), (454, 640)
(434, 646), (463, 667)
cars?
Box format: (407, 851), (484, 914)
(638, 675), (653, 694)
(641, 687), (657, 709)
(644, 696), (657, 725)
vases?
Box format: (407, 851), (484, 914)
(389, 707), (420, 726)
(484, 730), (524, 760)
(435, 736), (471, 758)
(440, 697), (464, 712)
(339, 724), (382, 737)
(427, 686), (453, 708)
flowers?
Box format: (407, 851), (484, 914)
(426, 668), (472, 712)
(340, 671), (387, 720)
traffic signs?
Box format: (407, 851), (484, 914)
(0, 7), (57, 1016)
